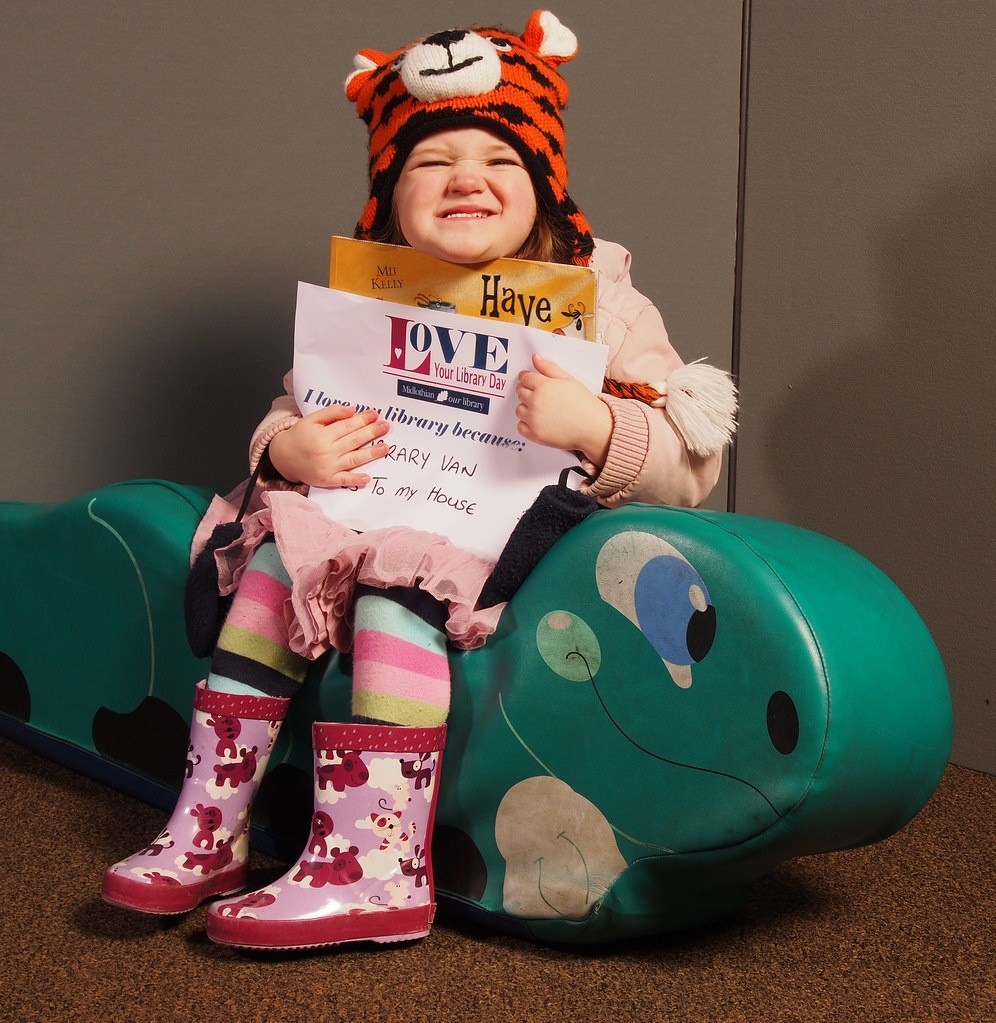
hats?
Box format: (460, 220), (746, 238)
(345, 9), (599, 268)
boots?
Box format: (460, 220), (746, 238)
(102, 676), (293, 914)
(208, 722), (450, 949)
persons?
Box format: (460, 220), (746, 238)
(96, 3), (745, 950)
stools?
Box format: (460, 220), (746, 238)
(0, 477), (957, 940)
